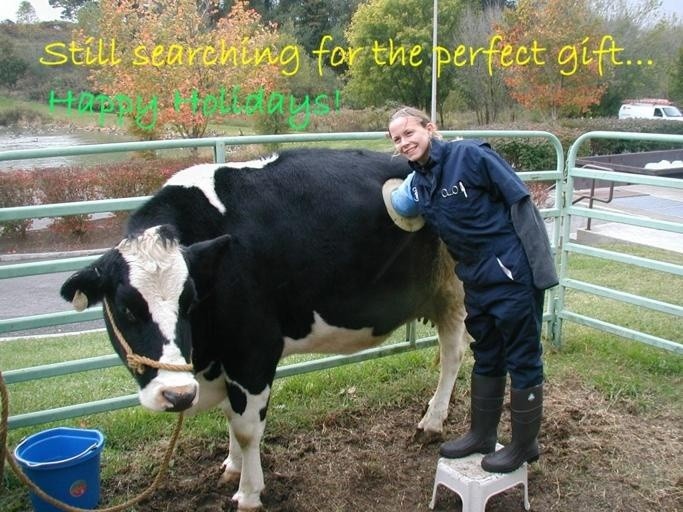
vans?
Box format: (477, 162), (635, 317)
(617, 99), (683, 123)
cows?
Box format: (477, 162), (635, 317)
(57, 145), (467, 512)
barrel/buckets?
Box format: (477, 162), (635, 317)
(13, 426), (106, 512)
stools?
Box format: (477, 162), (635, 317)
(426, 444), (530, 512)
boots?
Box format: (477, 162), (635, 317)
(439, 372), (507, 459)
(481, 383), (542, 472)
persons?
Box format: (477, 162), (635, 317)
(388, 107), (559, 473)
